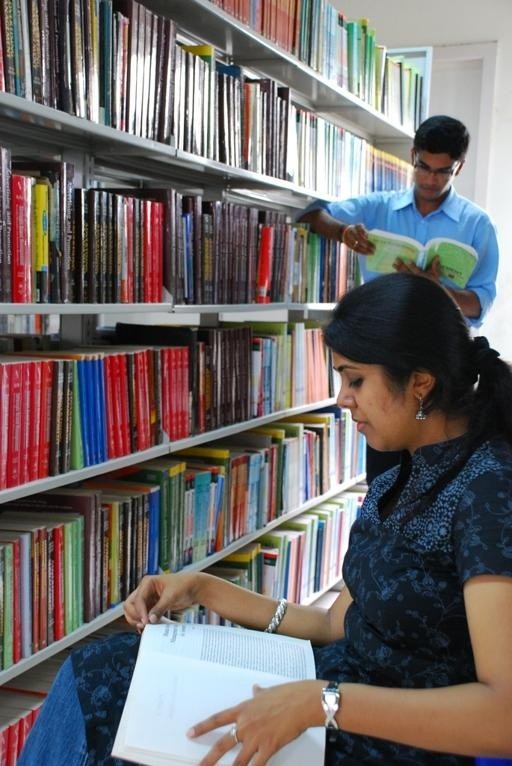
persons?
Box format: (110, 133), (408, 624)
(308, 112), (500, 485)
(8, 273), (512, 766)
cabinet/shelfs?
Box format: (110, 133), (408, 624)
(2, 0), (437, 763)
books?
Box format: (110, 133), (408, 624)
(0, 0), (423, 203)
(367, 228), (479, 288)
(1, 319), (370, 766)
(1, 143), (365, 306)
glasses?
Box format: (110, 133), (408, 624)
(411, 158), (458, 179)
